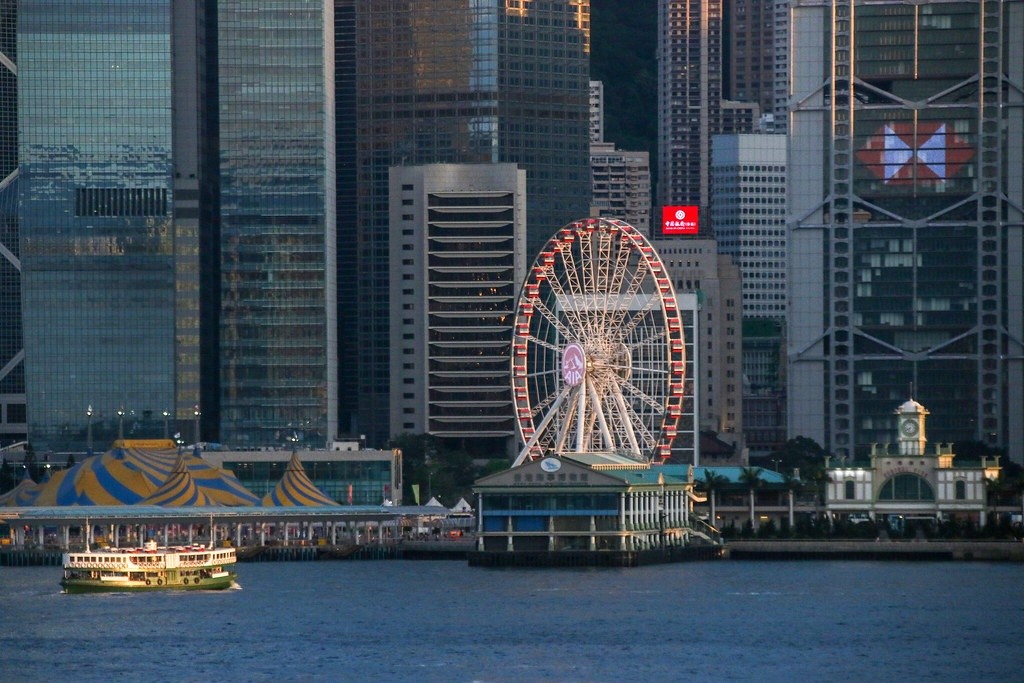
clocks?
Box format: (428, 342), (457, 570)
(901, 419), (919, 437)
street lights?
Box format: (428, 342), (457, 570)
(118, 411), (125, 439)
(194, 411), (201, 443)
(428, 471), (433, 499)
(86, 405), (93, 448)
(163, 412), (170, 439)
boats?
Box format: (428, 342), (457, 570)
(59, 540), (237, 594)
